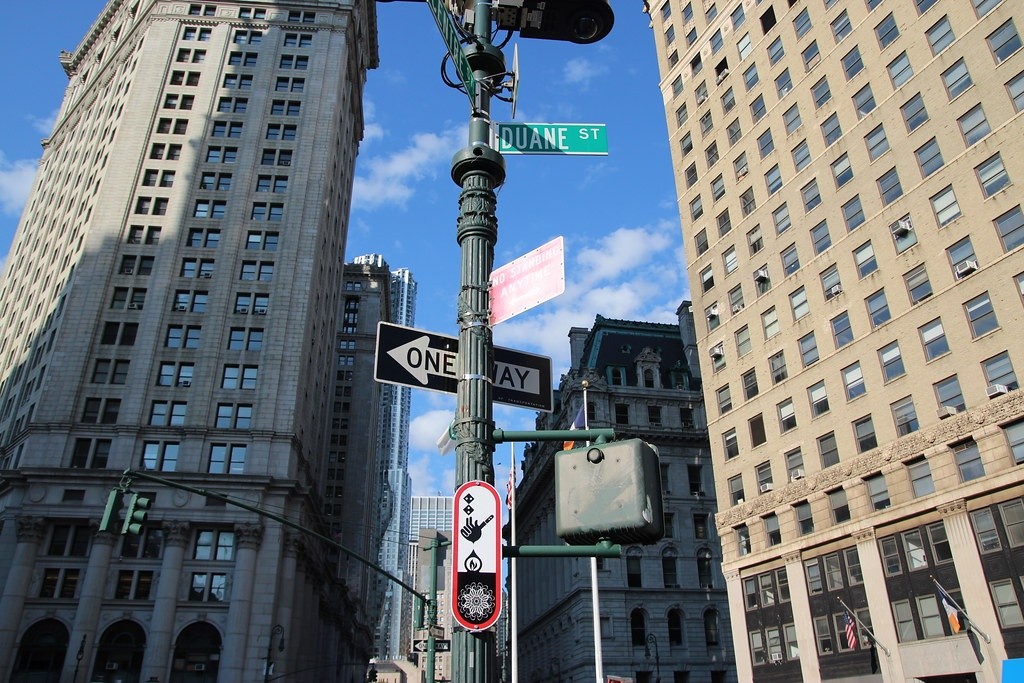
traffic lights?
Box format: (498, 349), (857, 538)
(367, 669), (378, 682)
(99, 488), (124, 537)
(122, 493), (151, 538)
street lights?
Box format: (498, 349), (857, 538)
(421, 0), (616, 682)
(644, 633), (662, 683)
(263, 624), (285, 683)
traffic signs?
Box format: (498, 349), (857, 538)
(427, 622), (444, 639)
(487, 236), (566, 329)
(426, 1), (478, 112)
(412, 639), (451, 653)
(374, 321), (555, 415)
(492, 120), (609, 157)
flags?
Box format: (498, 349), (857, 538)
(845, 609), (858, 650)
(936, 585), (962, 634)
